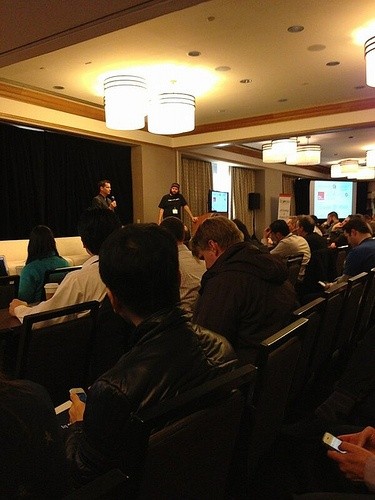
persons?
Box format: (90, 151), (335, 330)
(63, 222), (240, 477)
(310, 321), (375, 494)
(7, 216), (208, 330)
(190, 213), (302, 357)
(84, 180), (122, 235)
(1, 378), (82, 499)
(233, 210), (375, 301)
(158, 183), (197, 227)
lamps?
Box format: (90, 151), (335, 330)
(364, 36), (374, 87)
(262, 135), (323, 165)
(103, 74), (198, 133)
(331, 150), (375, 180)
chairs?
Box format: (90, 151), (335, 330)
(0, 240), (375, 499)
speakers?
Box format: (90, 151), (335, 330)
(248, 193), (260, 209)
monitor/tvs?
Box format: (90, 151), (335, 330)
(208, 190), (228, 213)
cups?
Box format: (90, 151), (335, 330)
(44, 284), (59, 301)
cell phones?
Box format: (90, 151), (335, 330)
(69, 388), (87, 403)
(322, 432), (348, 452)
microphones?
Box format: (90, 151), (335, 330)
(111, 196), (115, 202)
